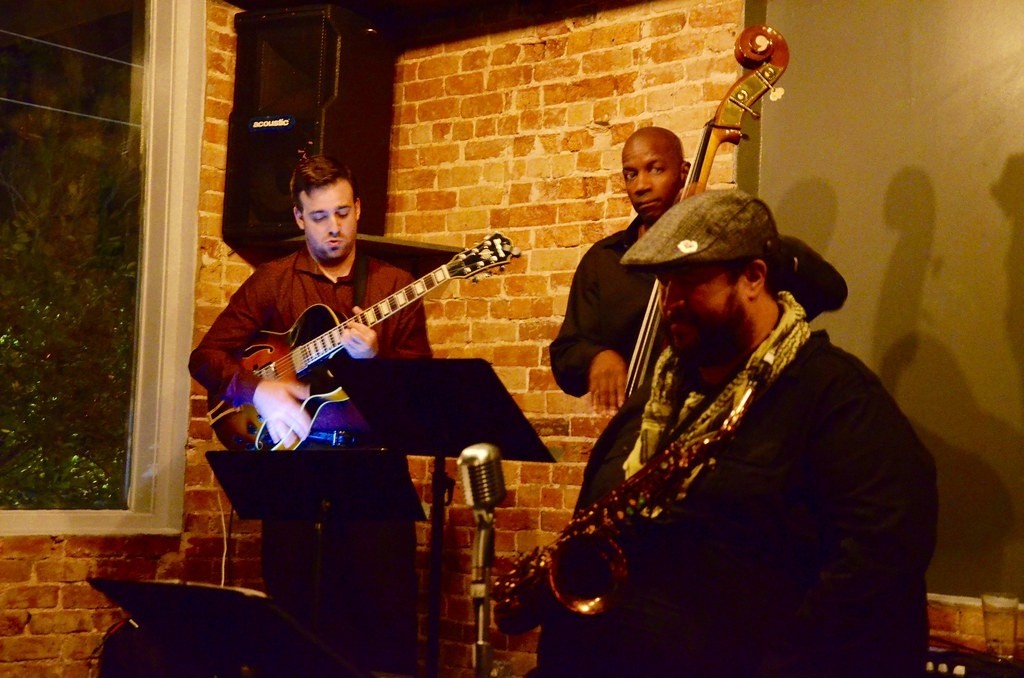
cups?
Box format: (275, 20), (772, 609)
(982, 592), (1019, 659)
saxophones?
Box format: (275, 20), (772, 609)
(494, 357), (774, 636)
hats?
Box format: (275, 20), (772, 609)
(618, 189), (779, 264)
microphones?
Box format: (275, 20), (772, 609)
(457, 443), (506, 510)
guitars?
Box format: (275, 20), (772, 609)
(207, 232), (513, 451)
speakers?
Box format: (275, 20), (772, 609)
(223, 2), (395, 248)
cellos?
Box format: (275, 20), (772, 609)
(622, 25), (791, 393)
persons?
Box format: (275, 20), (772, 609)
(189, 155), (433, 677)
(535, 190), (939, 678)
(549, 127), (849, 517)
